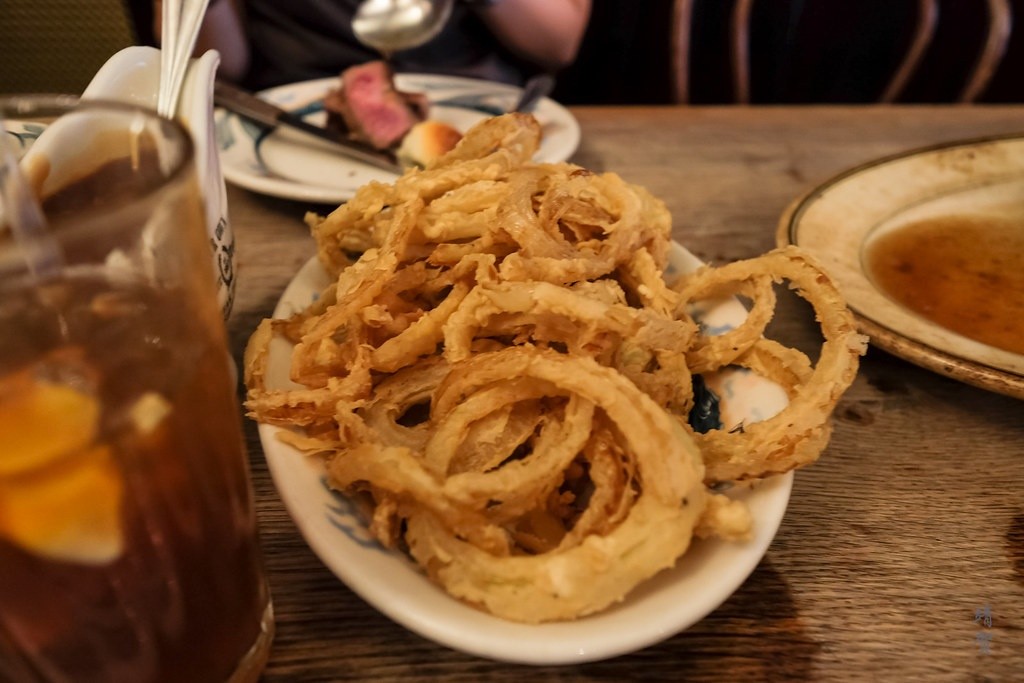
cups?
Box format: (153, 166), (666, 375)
(0, 95), (276, 683)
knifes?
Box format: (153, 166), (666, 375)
(214, 80), (400, 171)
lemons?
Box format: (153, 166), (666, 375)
(0, 344), (126, 567)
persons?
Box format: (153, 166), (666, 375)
(129, 0), (594, 99)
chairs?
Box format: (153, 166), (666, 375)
(672, 0), (1011, 107)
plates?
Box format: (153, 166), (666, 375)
(254, 238), (795, 665)
(214, 73), (580, 204)
(776, 134), (1024, 399)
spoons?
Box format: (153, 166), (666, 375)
(352, 1), (452, 53)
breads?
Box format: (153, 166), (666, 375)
(398, 121), (461, 165)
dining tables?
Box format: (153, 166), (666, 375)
(1, 108), (1024, 682)
